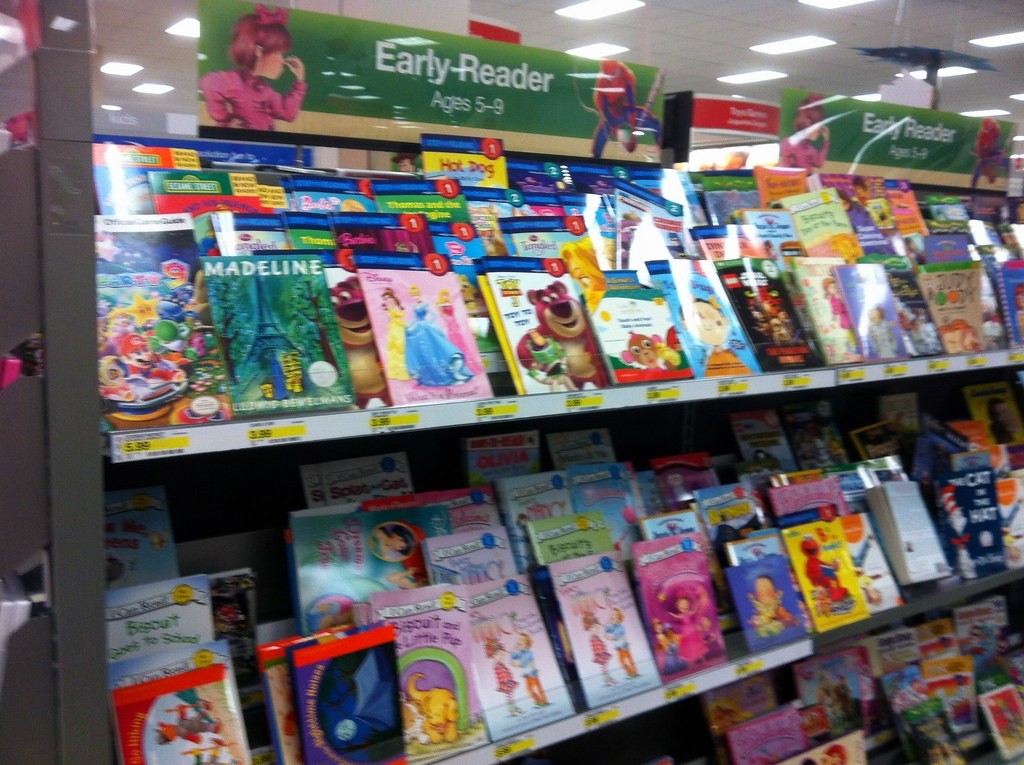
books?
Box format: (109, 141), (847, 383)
(102, 380), (1023, 765)
(90, 132), (1024, 434)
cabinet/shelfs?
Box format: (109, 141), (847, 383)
(0, 0), (1024, 765)
